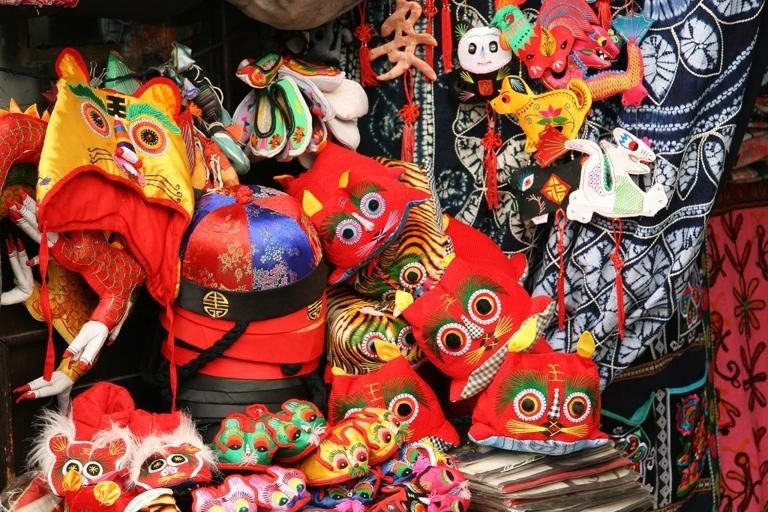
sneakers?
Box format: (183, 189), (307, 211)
(233, 52), (369, 170)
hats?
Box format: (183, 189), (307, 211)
(35, 49), (195, 307)
